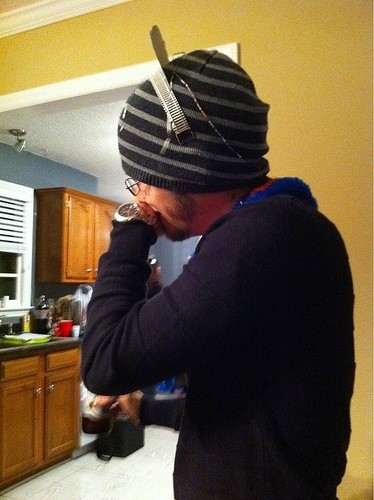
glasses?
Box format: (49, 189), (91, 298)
(125, 177), (140, 196)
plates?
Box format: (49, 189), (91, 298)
(1, 333), (51, 346)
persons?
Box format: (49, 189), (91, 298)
(81, 49), (355, 500)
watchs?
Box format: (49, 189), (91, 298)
(113, 201), (160, 235)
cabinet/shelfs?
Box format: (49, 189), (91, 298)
(34, 187), (122, 283)
(0, 348), (82, 491)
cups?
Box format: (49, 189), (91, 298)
(4, 296), (9, 308)
(60, 320), (73, 337)
(73, 325), (80, 337)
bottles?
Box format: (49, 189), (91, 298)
(35, 295), (50, 334)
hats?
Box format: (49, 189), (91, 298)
(118, 50), (270, 194)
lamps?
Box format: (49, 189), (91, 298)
(8, 128), (26, 152)
